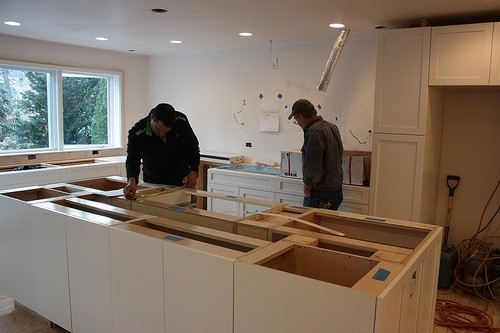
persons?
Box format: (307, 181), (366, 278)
(122, 102), (201, 205)
(288, 99), (344, 211)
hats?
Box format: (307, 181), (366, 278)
(288, 98), (314, 119)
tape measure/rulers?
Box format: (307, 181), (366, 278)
(135, 177), (188, 198)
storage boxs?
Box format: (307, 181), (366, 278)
(342, 149), (372, 185)
(277, 148), (303, 179)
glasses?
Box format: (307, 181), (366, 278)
(294, 116), (301, 126)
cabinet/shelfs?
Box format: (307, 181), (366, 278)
(0, 21), (500, 333)
